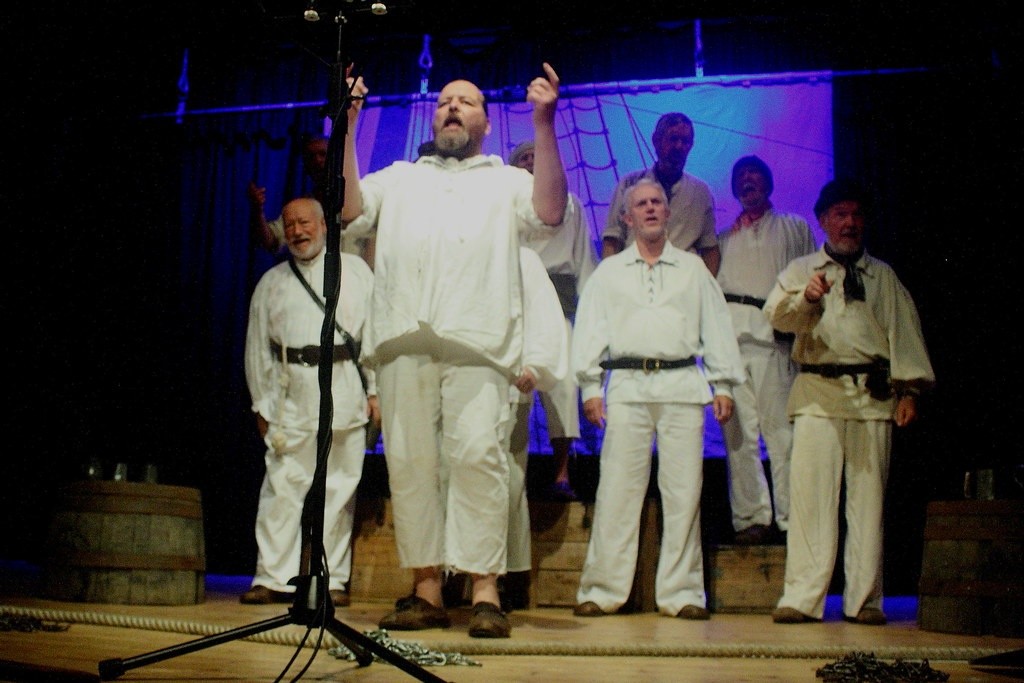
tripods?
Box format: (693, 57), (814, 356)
(94, 2), (451, 682)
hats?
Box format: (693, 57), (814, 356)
(814, 181), (867, 220)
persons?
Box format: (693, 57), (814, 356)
(245, 132), (372, 258)
(706, 154), (823, 547)
(600, 111), (721, 276)
(503, 138), (600, 315)
(336, 54), (572, 642)
(760, 179), (936, 623)
(235, 195), (386, 609)
(567, 176), (744, 619)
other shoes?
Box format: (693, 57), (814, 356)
(240, 584), (291, 605)
(467, 603), (510, 636)
(678, 604), (710, 621)
(379, 596), (450, 630)
(550, 483), (580, 501)
(772, 607), (814, 622)
(327, 590), (350, 606)
(574, 602), (604, 616)
(735, 525), (770, 544)
(842, 607), (888, 625)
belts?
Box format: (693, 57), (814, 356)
(724, 295), (766, 310)
(599, 356), (695, 375)
(268, 338), (361, 368)
(799, 361), (871, 379)
